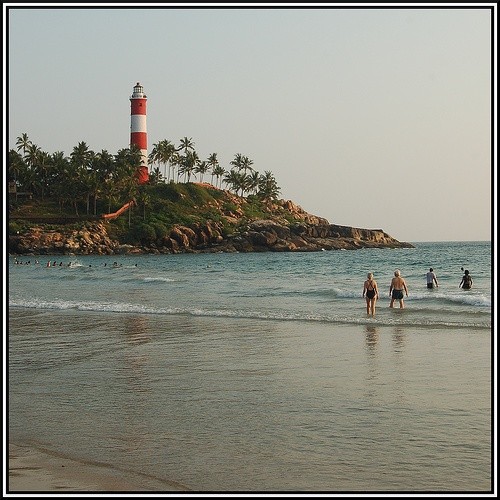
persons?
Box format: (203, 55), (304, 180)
(458, 269), (473, 290)
(427, 268), (439, 288)
(13, 256), (138, 268)
(389, 270), (407, 308)
(362, 272), (379, 315)
(461, 268), (464, 271)
